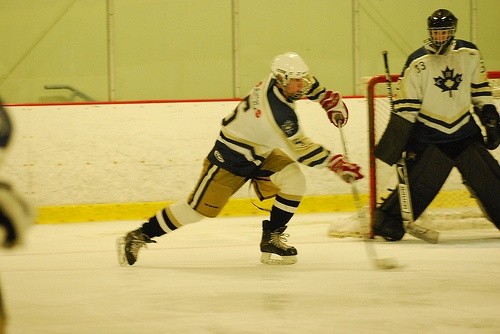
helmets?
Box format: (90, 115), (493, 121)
(426, 8), (458, 46)
(271, 52), (312, 98)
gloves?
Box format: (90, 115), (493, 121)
(326, 154), (364, 184)
(319, 91), (348, 128)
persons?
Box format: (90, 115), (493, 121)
(373, 9), (500, 243)
(117, 53), (367, 268)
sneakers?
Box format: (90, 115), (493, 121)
(116, 227), (157, 265)
(259, 220), (297, 265)
